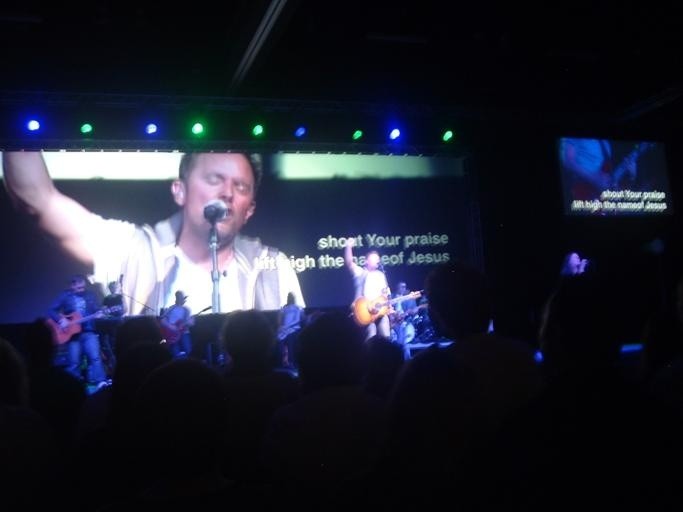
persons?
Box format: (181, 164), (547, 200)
(344, 239), (391, 342)
(560, 251), (589, 274)
(4, 151), (307, 316)
(1, 261), (683, 512)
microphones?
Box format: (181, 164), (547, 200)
(203, 199), (228, 222)
(64, 288), (75, 292)
(119, 275), (123, 283)
(377, 261), (386, 273)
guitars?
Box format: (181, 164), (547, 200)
(48, 310), (103, 345)
(350, 291), (422, 327)
(160, 306), (212, 344)
(275, 317), (305, 341)
